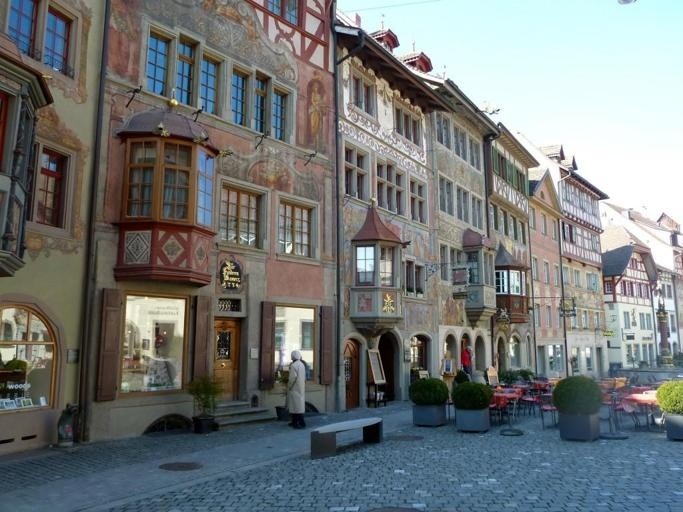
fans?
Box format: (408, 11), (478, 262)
(368, 350), (387, 384)
(486, 368), (499, 386)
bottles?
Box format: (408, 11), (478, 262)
(290, 351), (300, 360)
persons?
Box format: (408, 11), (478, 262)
(283, 350), (307, 430)
(461, 345), (474, 376)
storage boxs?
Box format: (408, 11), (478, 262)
(312, 417), (383, 459)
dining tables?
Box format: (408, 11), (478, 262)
(186, 370), (224, 434)
(275, 370), (293, 420)
(410, 377), (449, 427)
(552, 376), (604, 441)
(452, 381), (491, 433)
(657, 378), (683, 440)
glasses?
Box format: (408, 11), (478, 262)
(158, 121), (163, 130)
(202, 133), (209, 141)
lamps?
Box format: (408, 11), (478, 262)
(492, 374), (659, 430)
